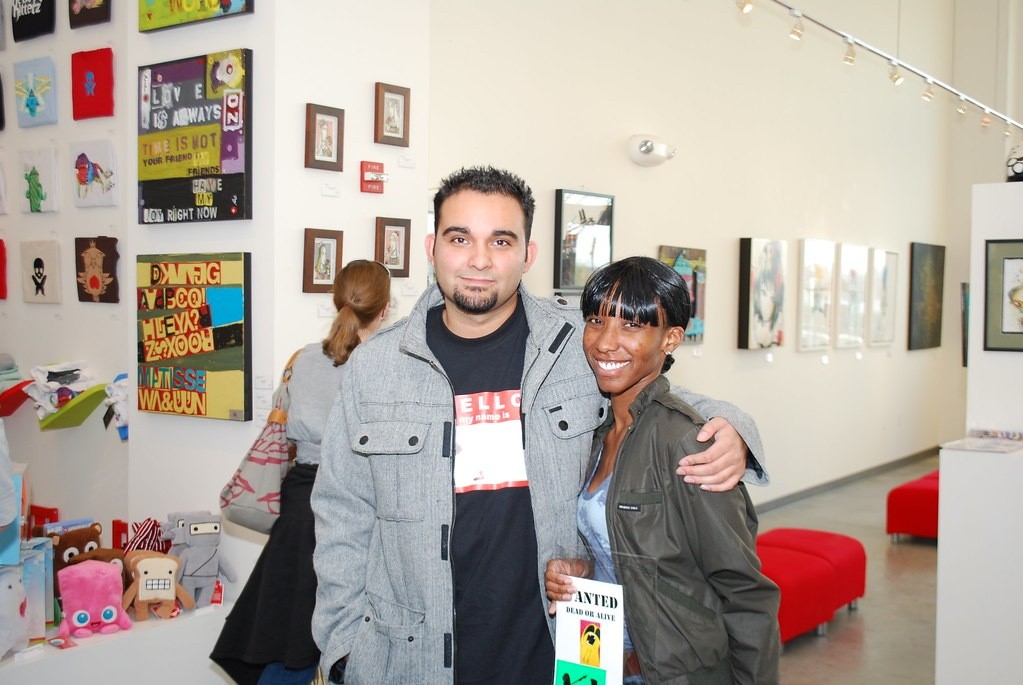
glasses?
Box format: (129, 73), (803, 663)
(353, 259), (391, 281)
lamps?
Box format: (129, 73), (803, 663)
(1003, 123), (1012, 135)
(921, 83), (937, 102)
(981, 113), (992, 128)
(789, 21), (805, 41)
(842, 44), (857, 65)
(735, 0), (754, 13)
(955, 99), (968, 113)
(887, 66), (905, 85)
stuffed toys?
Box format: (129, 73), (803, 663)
(48, 507), (239, 638)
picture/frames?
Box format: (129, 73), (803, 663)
(797, 238), (837, 351)
(553, 189), (614, 289)
(375, 216), (411, 276)
(865, 247), (899, 346)
(374, 81), (410, 146)
(302, 227), (343, 292)
(657, 245), (706, 345)
(908, 242), (946, 349)
(831, 241), (869, 348)
(960, 282), (969, 366)
(984, 238), (1023, 352)
(737, 237), (790, 349)
(305, 102), (345, 171)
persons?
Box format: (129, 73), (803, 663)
(541, 256), (781, 685)
(309, 164), (771, 685)
(247, 257), (399, 685)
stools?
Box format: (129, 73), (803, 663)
(886, 476), (939, 543)
(920, 467), (939, 478)
(755, 544), (836, 655)
(756, 527), (866, 612)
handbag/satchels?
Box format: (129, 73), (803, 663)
(218, 407), (297, 536)
